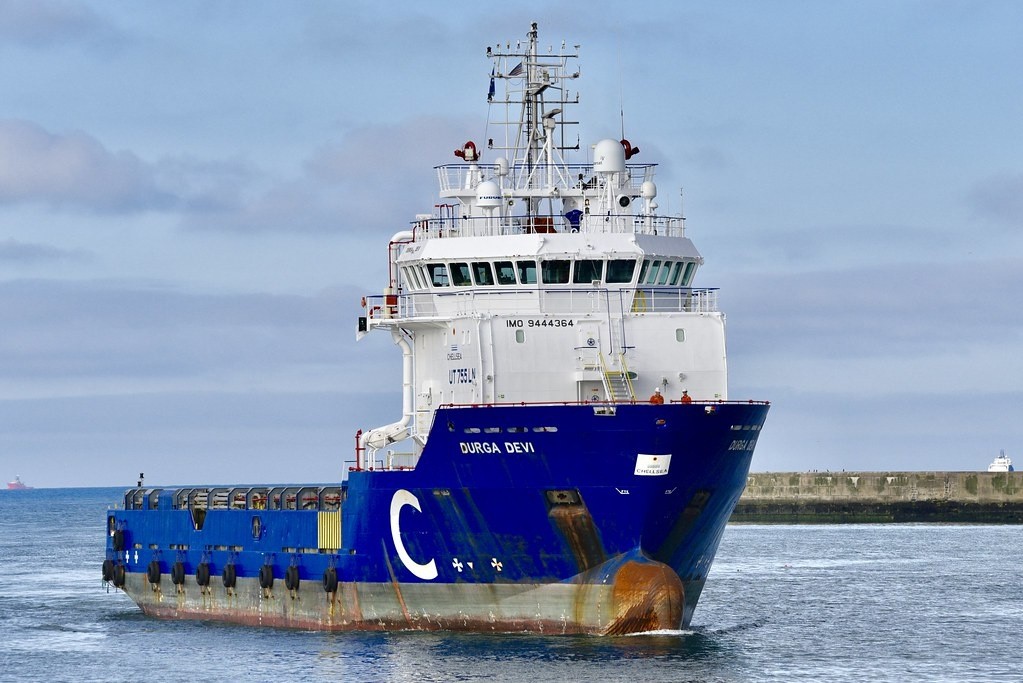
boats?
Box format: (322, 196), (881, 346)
(102, 21), (772, 636)
(6, 474), (34, 489)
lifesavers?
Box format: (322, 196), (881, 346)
(196, 563), (209, 586)
(171, 561), (185, 584)
(222, 565), (236, 587)
(259, 565), (273, 588)
(113, 531), (124, 551)
(370, 306), (383, 319)
(285, 566), (299, 590)
(323, 568), (337, 592)
(102, 560), (113, 581)
(147, 561), (160, 583)
(112, 565), (124, 587)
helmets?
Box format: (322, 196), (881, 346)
(682, 389), (687, 392)
(655, 388), (660, 393)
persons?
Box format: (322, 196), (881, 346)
(681, 389), (691, 404)
(650, 388), (664, 404)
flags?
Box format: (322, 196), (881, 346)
(489, 69), (495, 96)
(509, 63), (522, 76)
